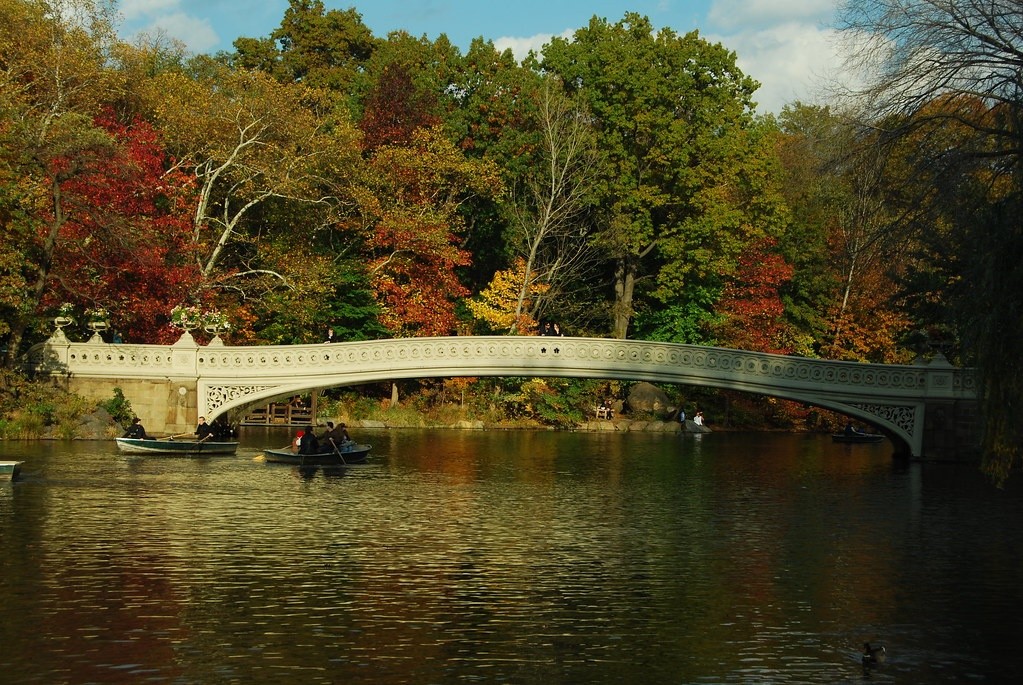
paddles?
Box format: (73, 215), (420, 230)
(331, 440), (347, 465)
(190, 435), (210, 450)
(161, 432), (193, 439)
(282, 445), (293, 449)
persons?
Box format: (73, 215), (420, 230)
(681, 410), (686, 429)
(693, 412), (704, 426)
(121, 418), (156, 440)
(844, 418), (865, 436)
(323, 328), (337, 343)
(193, 416), (216, 442)
(539, 321), (567, 336)
(292, 421), (350, 455)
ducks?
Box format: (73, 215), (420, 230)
(862, 642), (885, 665)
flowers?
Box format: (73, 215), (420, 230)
(81, 308), (112, 329)
(926, 327), (956, 345)
(168, 303), (232, 333)
(901, 330), (930, 345)
(42, 302), (80, 326)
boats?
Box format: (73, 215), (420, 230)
(262, 444), (372, 466)
(832, 431), (886, 443)
(115, 432), (241, 455)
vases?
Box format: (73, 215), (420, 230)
(910, 344), (929, 351)
(49, 316), (70, 322)
(206, 324), (230, 330)
(926, 340), (953, 345)
(174, 321), (195, 325)
(87, 322), (106, 327)
